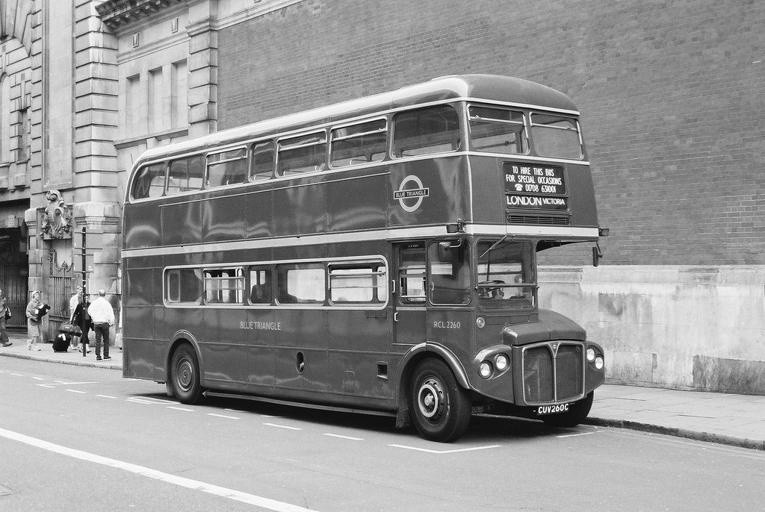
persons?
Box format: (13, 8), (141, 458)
(0, 288), (13, 347)
(86, 288), (117, 362)
(25, 291), (51, 352)
(250, 284), (270, 302)
(68, 285), (83, 351)
(70, 294), (94, 353)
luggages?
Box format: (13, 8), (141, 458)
(52, 333), (72, 353)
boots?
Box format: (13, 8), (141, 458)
(86, 344), (91, 353)
(79, 343), (83, 353)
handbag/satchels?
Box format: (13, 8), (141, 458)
(4, 307), (12, 320)
(60, 321), (83, 337)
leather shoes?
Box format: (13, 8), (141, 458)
(27, 342), (42, 351)
(96, 354), (111, 360)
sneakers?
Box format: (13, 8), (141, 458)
(2, 342), (13, 347)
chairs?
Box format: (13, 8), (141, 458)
(424, 274), (461, 302)
(251, 282), (297, 303)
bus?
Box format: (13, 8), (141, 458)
(119, 73), (606, 445)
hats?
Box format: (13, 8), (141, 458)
(32, 290), (41, 299)
